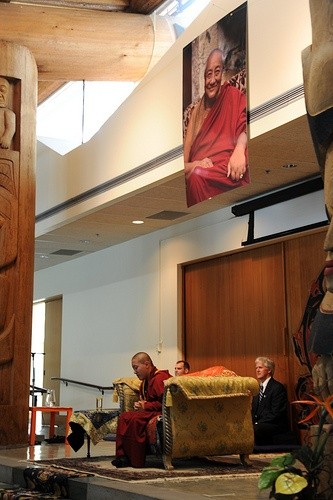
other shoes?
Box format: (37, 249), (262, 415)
(111, 455), (130, 466)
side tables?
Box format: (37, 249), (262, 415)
(67, 408), (119, 457)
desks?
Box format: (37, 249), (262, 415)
(29, 406), (73, 446)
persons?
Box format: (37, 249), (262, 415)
(250, 357), (302, 447)
(183, 48), (249, 208)
(111, 352), (173, 469)
(0, 77), (17, 150)
(301, 2), (333, 407)
(175, 360), (189, 376)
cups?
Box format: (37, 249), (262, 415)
(96, 397), (103, 411)
(45, 389), (56, 407)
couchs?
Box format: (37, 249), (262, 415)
(111, 366), (259, 470)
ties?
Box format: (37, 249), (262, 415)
(260, 385), (263, 402)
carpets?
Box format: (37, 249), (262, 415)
(18, 455), (276, 484)
